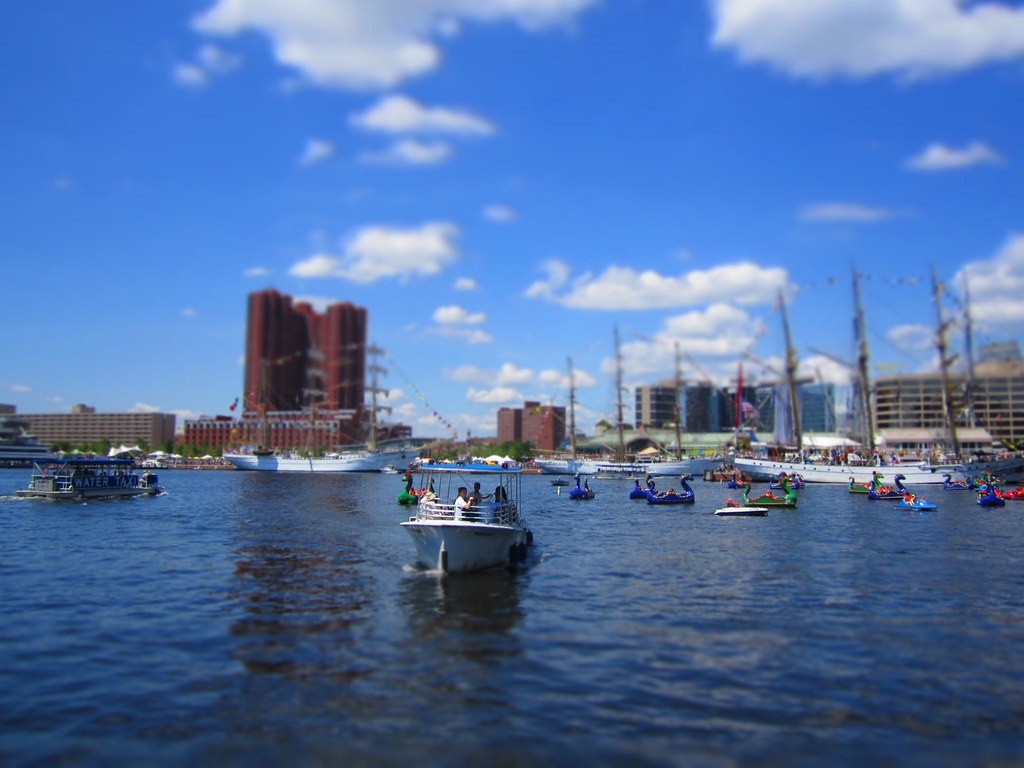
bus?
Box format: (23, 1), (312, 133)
(664, 445), (725, 459)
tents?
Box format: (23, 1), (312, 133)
(502, 455), (516, 467)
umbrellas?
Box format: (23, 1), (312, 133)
(148, 450), (182, 460)
(106, 444), (143, 459)
(186, 454), (213, 465)
(57, 449), (97, 460)
(485, 454), (503, 462)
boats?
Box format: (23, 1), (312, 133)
(743, 472), (802, 508)
(868, 474), (910, 500)
(974, 472), (1024, 506)
(523, 464), (544, 473)
(848, 473), (884, 493)
(416, 462), (523, 474)
(400, 464), (533, 573)
(398, 471), (438, 506)
(769, 472), (805, 489)
(379, 464), (398, 475)
(630, 473), (658, 499)
(15, 458), (165, 499)
(551, 477), (569, 486)
(583, 476), (595, 498)
(894, 497), (937, 511)
(728, 472), (743, 489)
(942, 473), (973, 490)
(714, 499), (769, 516)
(737, 473), (751, 488)
(569, 472), (587, 498)
(647, 472), (695, 505)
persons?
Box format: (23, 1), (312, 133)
(43, 465), (124, 476)
(453, 487), (475, 522)
(495, 486), (507, 503)
(465, 482), (482, 509)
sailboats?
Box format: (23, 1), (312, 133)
(223, 342), (421, 472)
(647, 343), (721, 478)
(733, 267), (1024, 484)
(535, 360), (609, 475)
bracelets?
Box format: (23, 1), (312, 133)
(469, 500), (472, 502)
